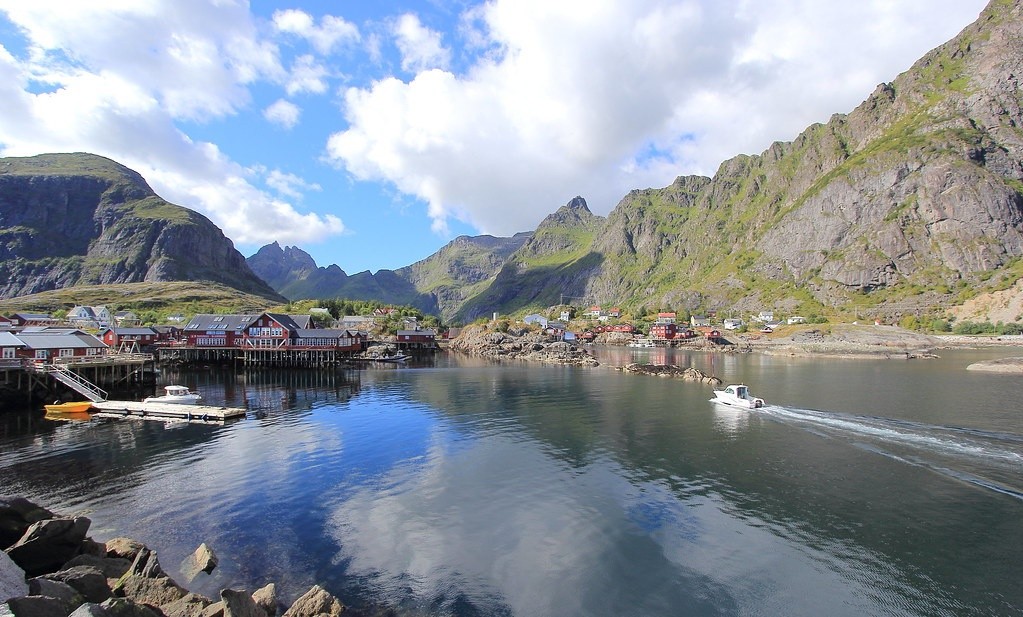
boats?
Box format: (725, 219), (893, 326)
(760, 327), (773, 333)
(713, 383), (765, 410)
(135, 366), (161, 375)
(145, 385), (201, 406)
(392, 350), (410, 361)
(44, 399), (91, 413)
(163, 420), (188, 427)
(628, 339), (656, 348)
